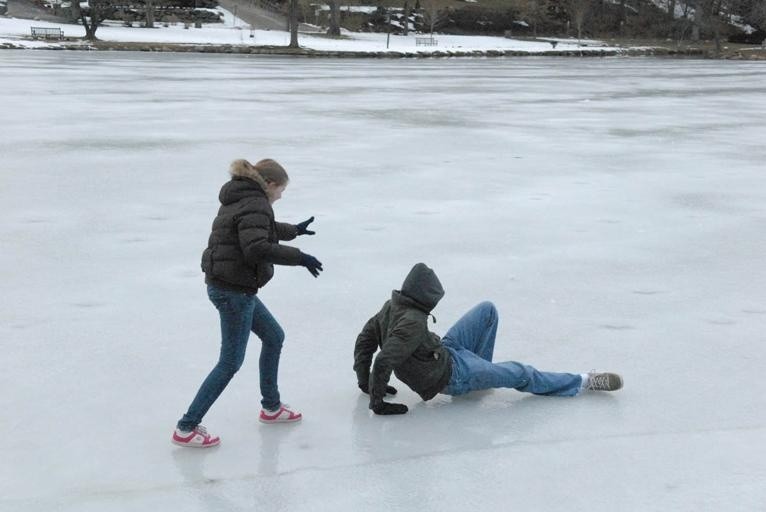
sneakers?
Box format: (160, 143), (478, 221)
(583, 369), (625, 392)
(171, 425), (222, 447)
(258, 405), (303, 422)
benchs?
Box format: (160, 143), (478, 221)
(416, 37), (437, 46)
(30, 26), (62, 39)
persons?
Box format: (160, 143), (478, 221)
(352, 261), (623, 413)
(173, 157), (325, 446)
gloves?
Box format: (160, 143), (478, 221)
(295, 216), (316, 237)
(360, 383), (397, 397)
(302, 253), (324, 278)
(373, 401), (409, 416)
